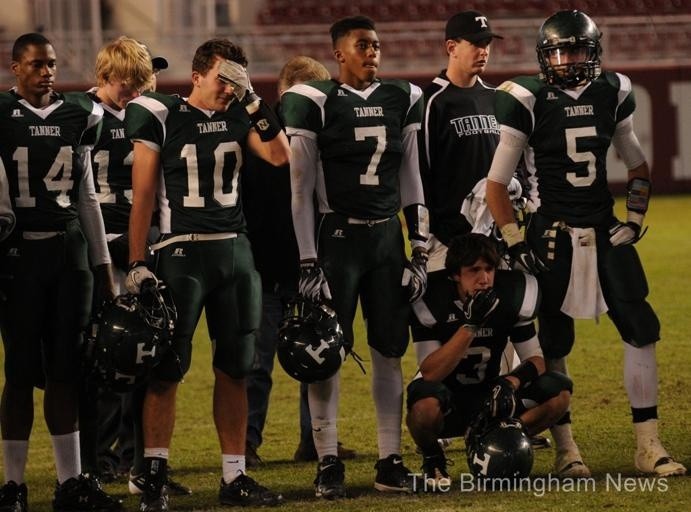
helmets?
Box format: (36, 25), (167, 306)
(466, 418), (534, 489)
(536, 10), (602, 89)
(80, 279), (177, 392)
(277, 292), (345, 382)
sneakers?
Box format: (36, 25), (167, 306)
(634, 449), (687, 477)
(0, 439), (451, 512)
(558, 449), (591, 477)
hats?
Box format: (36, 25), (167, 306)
(446, 12), (503, 42)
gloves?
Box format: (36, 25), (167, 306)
(609, 223), (640, 246)
(481, 378), (516, 419)
(500, 223), (550, 274)
(461, 287), (499, 328)
(218, 61), (261, 113)
(402, 250), (429, 302)
(299, 258), (332, 302)
(124, 260), (158, 293)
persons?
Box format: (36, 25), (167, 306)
(405, 233), (573, 490)
(0, 32), (119, 512)
(125, 39), (291, 512)
(277, 15), (429, 500)
(245, 56), (358, 471)
(484, 10), (687, 481)
(418, 12), (553, 451)
(144, 55), (169, 94)
(63, 37), (172, 486)
